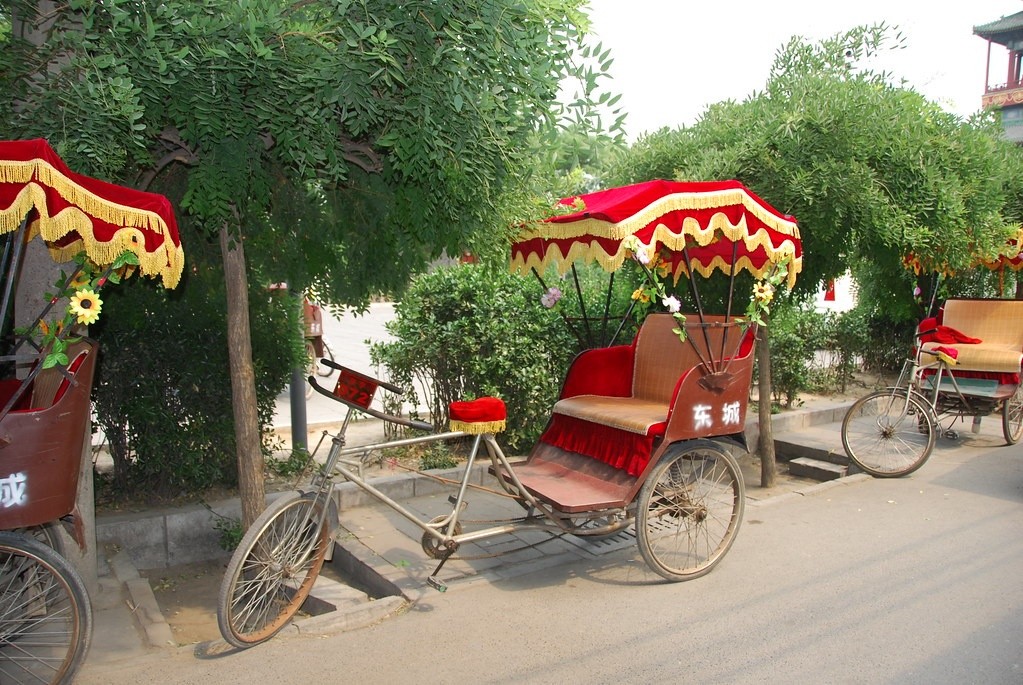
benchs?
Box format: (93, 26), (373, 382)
(919, 297), (1023, 373)
(0, 336), (92, 414)
(552, 314), (754, 437)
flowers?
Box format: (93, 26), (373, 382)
(631, 283), (650, 323)
(42, 252), (141, 369)
(13, 252), (93, 347)
(540, 287), (567, 312)
(734, 249), (792, 335)
(662, 295), (682, 313)
(625, 236), (651, 265)
(912, 281), (922, 304)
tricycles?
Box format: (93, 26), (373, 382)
(0, 136), (186, 685)
(840, 227), (1023, 478)
(215, 180), (804, 651)
